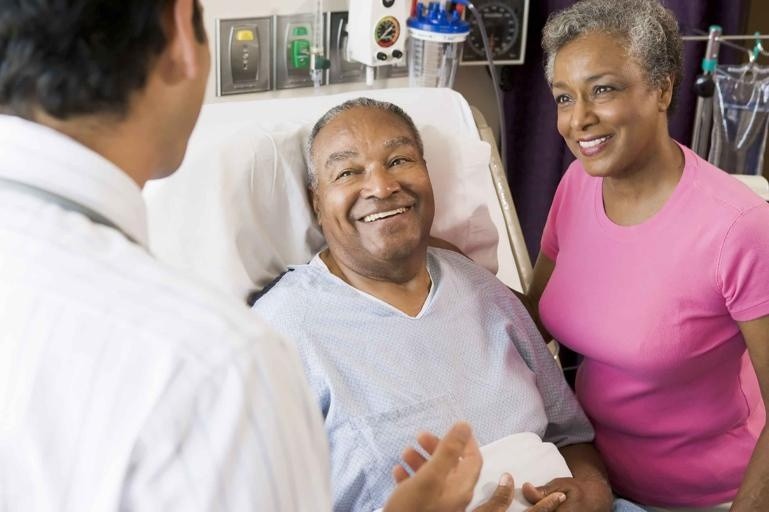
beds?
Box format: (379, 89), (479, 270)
(145, 88), (633, 506)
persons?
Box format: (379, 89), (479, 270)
(250, 95), (618, 512)
(427, 0), (768, 512)
(0, 0), (488, 512)
